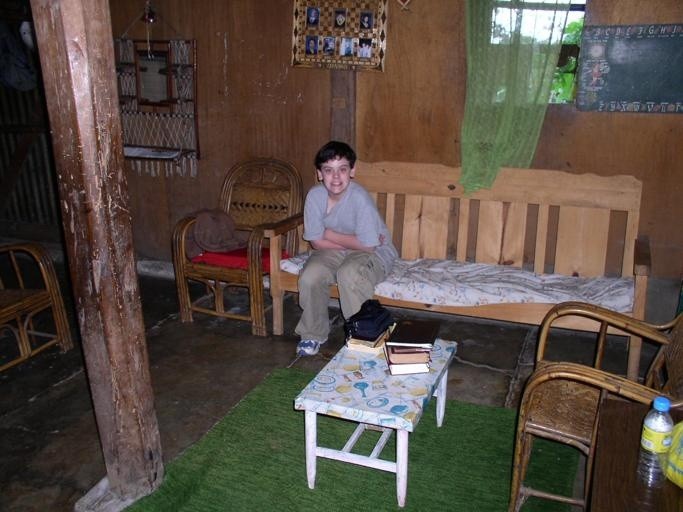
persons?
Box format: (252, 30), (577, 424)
(292, 140), (400, 358)
(304, 8), (372, 58)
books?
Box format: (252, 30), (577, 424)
(346, 308), (441, 377)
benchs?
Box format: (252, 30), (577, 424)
(262, 159), (650, 381)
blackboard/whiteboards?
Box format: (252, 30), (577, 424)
(577, 22), (683, 115)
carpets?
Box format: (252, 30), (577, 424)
(120, 365), (580, 512)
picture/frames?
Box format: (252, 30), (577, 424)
(290, 0), (388, 72)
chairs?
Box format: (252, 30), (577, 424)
(170, 156), (303, 337)
(507, 300), (681, 511)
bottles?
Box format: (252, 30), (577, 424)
(634, 397), (674, 490)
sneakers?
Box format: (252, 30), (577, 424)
(296, 334), (331, 358)
